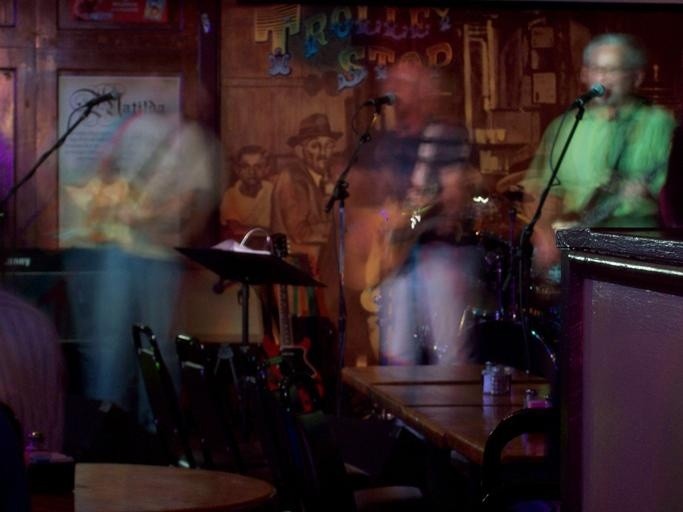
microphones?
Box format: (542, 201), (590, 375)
(86, 89), (119, 106)
(361, 93), (397, 107)
(570, 82), (606, 108)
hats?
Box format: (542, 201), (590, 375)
(286, 113), (343, 147)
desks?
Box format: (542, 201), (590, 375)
(76, 464), (277, 512)
(340, 365), (564, 477)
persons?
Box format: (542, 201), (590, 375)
(82, 76), (225, 399)
(217, 140), (270, 230)
(344, 55), (485, 367)
(268, 106), (340, 323)
(513, 27), (677, 275)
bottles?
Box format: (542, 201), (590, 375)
(481, 361), (511, 394)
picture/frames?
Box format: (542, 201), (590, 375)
(57, 69), (182, 249)
